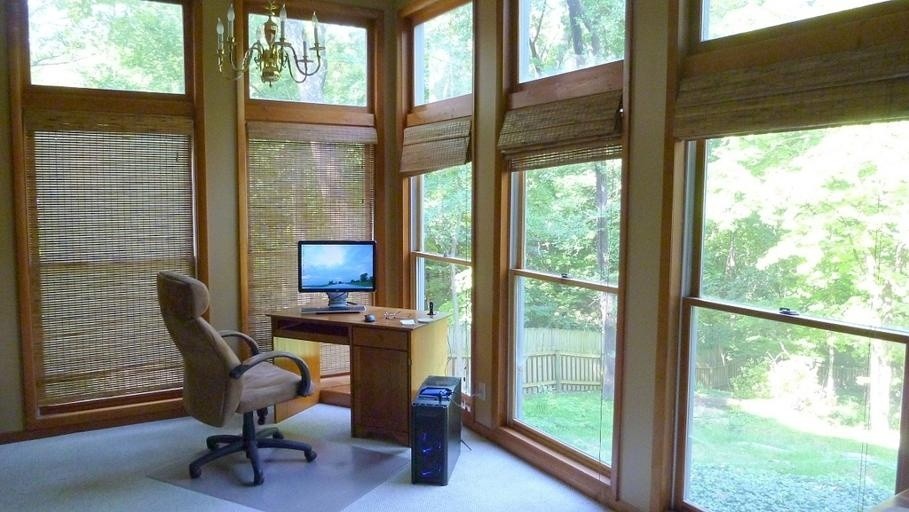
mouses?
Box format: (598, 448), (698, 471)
(364, 314), (376, 322)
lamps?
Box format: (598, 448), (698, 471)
(215, 2), (323, 86)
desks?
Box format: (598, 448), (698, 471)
(256, 299), (447, 445)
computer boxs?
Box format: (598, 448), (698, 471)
(409, 375), (462, 486)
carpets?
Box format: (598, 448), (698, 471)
(145, 429), (408, 511)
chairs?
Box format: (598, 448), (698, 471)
(156, 272), (318, 488)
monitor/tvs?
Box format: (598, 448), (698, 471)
(297, 240), (377, 307)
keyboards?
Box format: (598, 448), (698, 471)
(300, 305), (367, 313)
